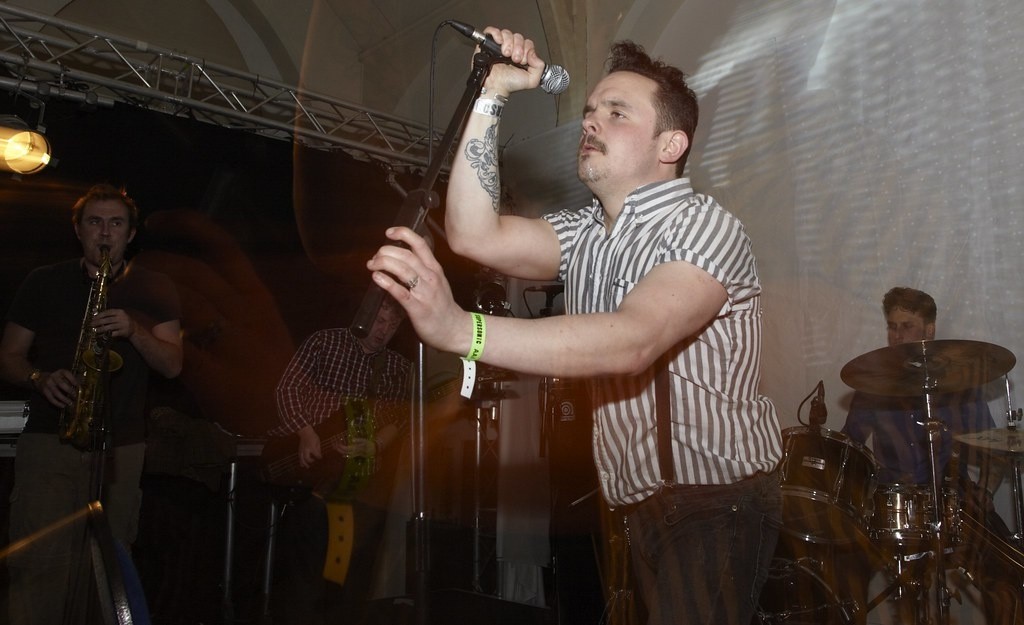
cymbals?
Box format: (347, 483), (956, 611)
(952, 429), (1024, 455)
(841, 334), (1017, 401)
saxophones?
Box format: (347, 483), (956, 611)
(60, 242), (125, 445)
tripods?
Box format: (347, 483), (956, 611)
(866, 375), (1024, 625)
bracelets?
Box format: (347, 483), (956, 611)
(461, 312), (486, 399)
(977, 485), (993, 497)
(473, 88), (508, 118)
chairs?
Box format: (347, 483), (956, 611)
(86, 511), (152, 624)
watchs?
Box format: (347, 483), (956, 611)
(27, 368), (40, 389)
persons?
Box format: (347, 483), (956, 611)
(0, 185), (183, 625)
(839, 287), (1024, 625)
(366, 27), (784, 625)
(276, 291), (413, 625)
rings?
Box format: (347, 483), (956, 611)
(406, 275), (420, 289)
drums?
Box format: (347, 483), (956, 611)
(752, 551), (873, 625)
(874, 481), (963, 555)
(766, 426), (887, 546)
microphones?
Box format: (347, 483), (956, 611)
(817, 381), (828, 424)
(451, 21), (570, 95)
(526, 284), (564, 293)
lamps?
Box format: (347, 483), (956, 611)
(0, 85), (51, 176)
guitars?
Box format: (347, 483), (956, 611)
(261, 366), (517, 502)
